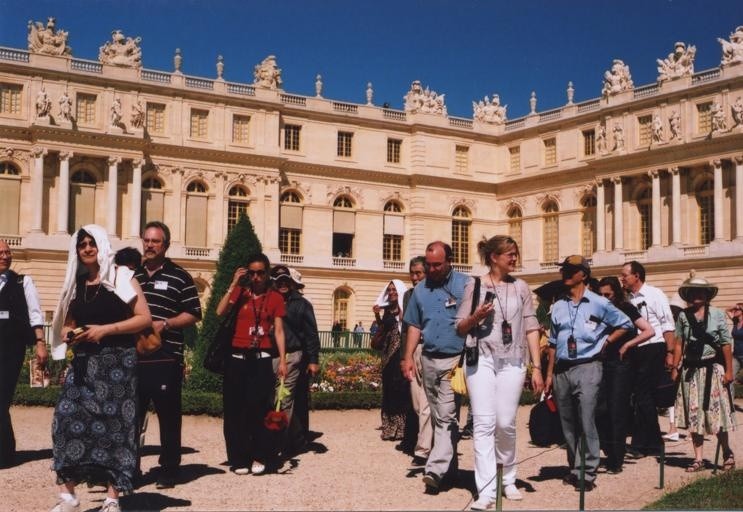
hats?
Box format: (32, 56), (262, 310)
(554, 255), (591, 274)
(288, 268), (305, 289)
(270, 265), (290, 281)
(679, 277), (718, 301)
(731, 303), (743, 310)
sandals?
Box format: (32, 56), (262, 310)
(686, 460), (706, 472)
(723, 450), (735, 470)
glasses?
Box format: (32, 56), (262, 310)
(78, 240), (96, 249)
(598, 292), (614, 298)
(248, 270), (266, 275)
(1, 250), (13, 258)
(560, 267), (580, 273)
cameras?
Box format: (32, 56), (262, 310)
(67, 327), (87, 344)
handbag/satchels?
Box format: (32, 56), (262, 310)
(447, 363), (468, 397)
(265, 411), (289, 432)
(529, 397), (566, 446)
(371, 327), (387, 349)
(203, 324), (232, 374)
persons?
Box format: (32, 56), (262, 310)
(0, 239), (48, 468)
(330, 322), (365, 348)
(51, 223), (152, 512)
(216, 255), (320, 475)
(372, 234), (743, 511)
(114, 245), (140, 269)
(135, 221), (202, 487)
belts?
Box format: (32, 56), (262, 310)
(422, 350), (461, 359)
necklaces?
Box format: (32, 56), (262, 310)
(84, 272), (102, 303)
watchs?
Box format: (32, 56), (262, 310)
(36, 337), (46, 342)
(163, 318), (171, 334)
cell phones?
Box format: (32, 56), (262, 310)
(484, 292), (495, 304)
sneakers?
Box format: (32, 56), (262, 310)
(407, 457), (427, 469)
(501, 484), (522, 500)
(102, 500), (121, 512)
(662, 432), (679, 440)
(156, 464), (180, 489)
(563, 472), (595, 491)
(625, 447), (648, 458)
(49, 498), (80, 512)
(423, 473), (440, 489)
(234, 464), (267, 474)
(471, 496), (496, 511)
